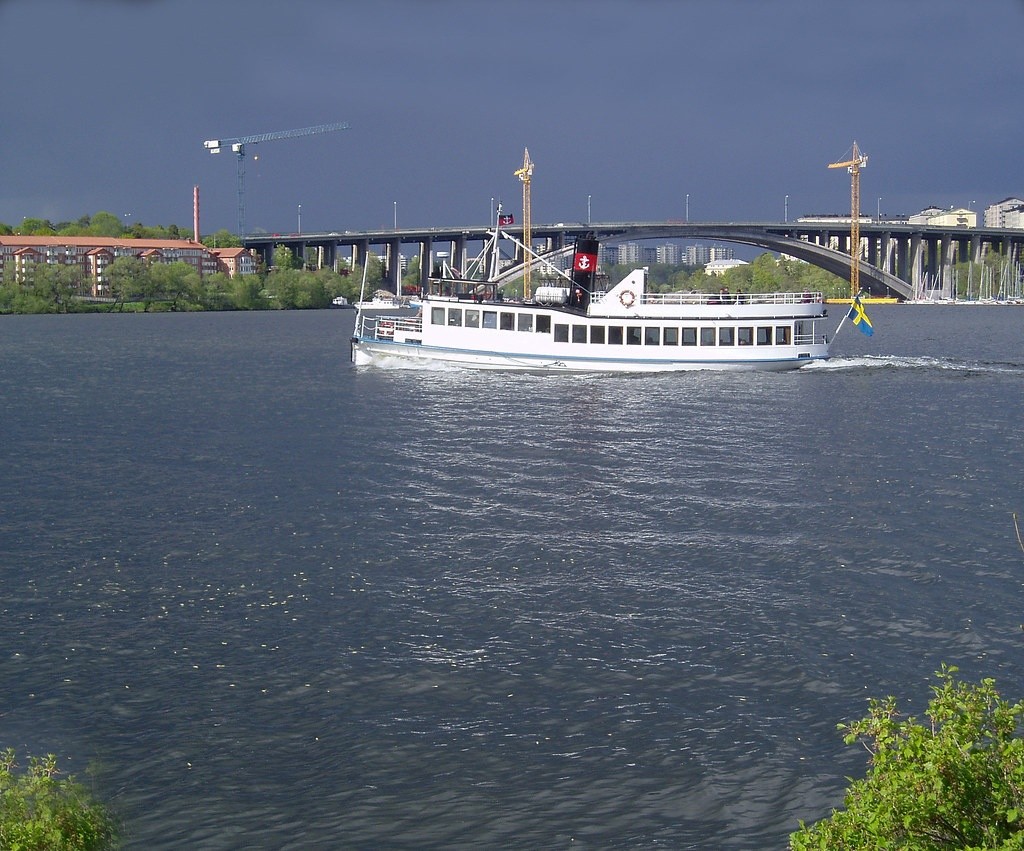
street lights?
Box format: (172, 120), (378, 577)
(297, 205), (302, 235)
(785, 194), (788, 222)
(968, 201), (976, 212)
(877, 198), (882, 223)
(587, 195), (592, 223)
(125, 212), (131, 231)
(491, 197), (494, 225)
(686, 194), (689, 220)
(393, 202), (396, 230)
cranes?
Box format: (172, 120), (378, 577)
(512, 147), (536, 300)
(828, 139), (869, 298)
(201, 120), (352, 248)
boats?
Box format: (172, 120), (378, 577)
(329, 296), (356, 309)
(349, 202), (832, 373)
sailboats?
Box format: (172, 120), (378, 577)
(898, 259), (1024, 306)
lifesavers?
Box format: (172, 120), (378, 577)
(380, 321), (394, 335)
(618, 290), (636, 307)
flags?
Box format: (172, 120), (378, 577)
(846, 294), (875, 338)
(498, 213), (516, 226)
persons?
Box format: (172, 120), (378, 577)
(800, 286), (821, 303)
(719, 287), (746, 305)
